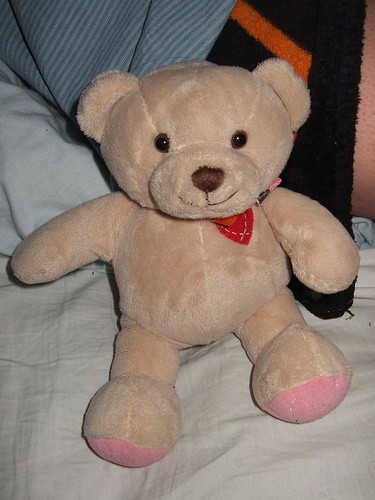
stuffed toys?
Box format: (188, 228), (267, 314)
(11, 59), (361, 468)
